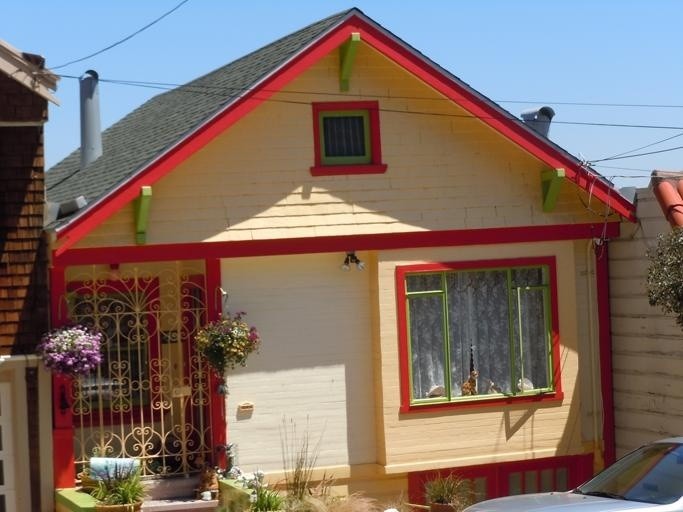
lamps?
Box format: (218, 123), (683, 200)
(342, 256), (368, 272)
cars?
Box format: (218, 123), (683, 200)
(457, 438), (683, 512)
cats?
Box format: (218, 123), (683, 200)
(461, 370), (480, 397)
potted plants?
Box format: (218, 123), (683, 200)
(77, 456), (151, 512)
(420, 470), (486, 511)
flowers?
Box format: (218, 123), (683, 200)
(34, 317), (104, 376)
(189, 311), (261, 370)
